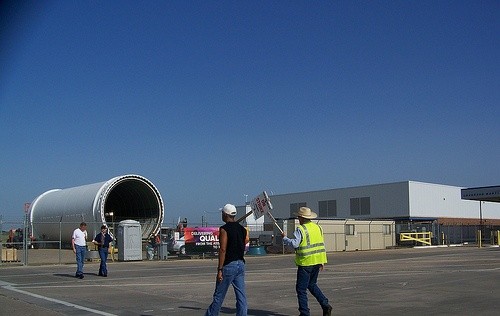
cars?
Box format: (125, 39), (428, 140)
(167, 236), (185, 254)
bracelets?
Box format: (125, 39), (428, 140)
(219, 269), (222, 270)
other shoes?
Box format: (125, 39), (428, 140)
(76, 272), (79, 277)
(323, 304), (332, 316)
(80, 275), (84, 279)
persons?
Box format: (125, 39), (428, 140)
(280, 207), (332, 316)
(94, 226), (112, 277)
(72, 223), (87, 279)
(205, 204), (250, 316)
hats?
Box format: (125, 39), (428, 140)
(219, 204), (237, 216)
(297, 207), (317, 219)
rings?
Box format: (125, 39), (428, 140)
(220, 279), (221, 280)
